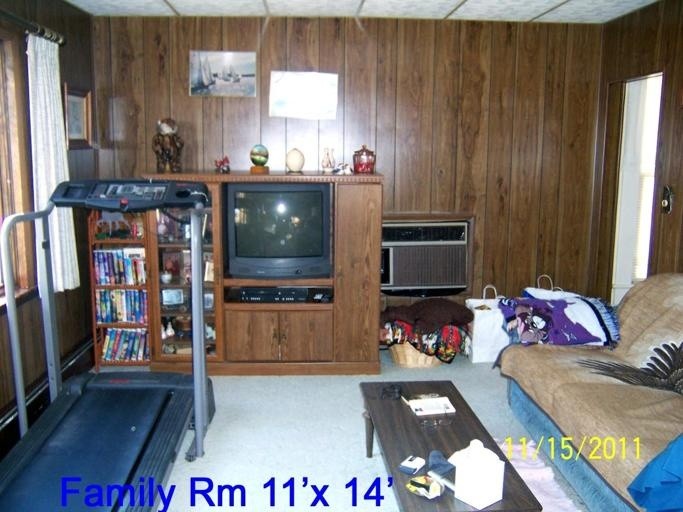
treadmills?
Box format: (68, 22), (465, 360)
(0, 180), (215, 512)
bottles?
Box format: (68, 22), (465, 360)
(160, 321), (175, 346)
(353, 145), (376, 173)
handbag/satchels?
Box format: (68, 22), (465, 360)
(466, 285), (510, 365)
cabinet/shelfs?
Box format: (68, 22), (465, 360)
(88, 175), (382, 377)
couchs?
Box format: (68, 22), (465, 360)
(508, 272), (683, 510)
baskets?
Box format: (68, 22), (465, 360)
(389, 342), (442, 369)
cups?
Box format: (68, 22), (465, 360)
(162, 270), (172, 284)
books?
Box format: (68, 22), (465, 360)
(94, 249), (149, 362)
(408, 396), (456, 416)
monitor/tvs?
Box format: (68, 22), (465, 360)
(221, 182), (331, 278)
